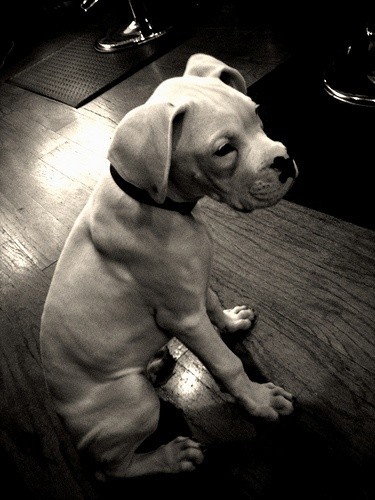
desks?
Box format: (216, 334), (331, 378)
(2, 1), (375, 500)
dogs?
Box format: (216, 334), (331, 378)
(38, 53), (301, 484)
